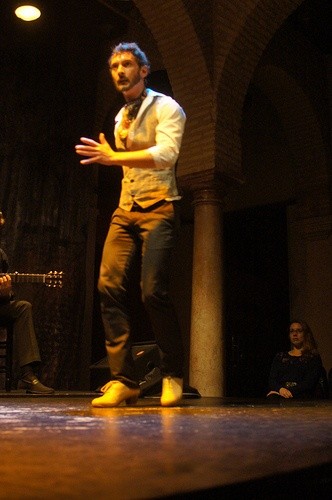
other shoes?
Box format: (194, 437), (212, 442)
(16, 378), (55, 395)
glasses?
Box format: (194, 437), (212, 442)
(289, 329), (304, 333)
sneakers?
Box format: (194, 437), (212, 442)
(160, 376), (184, 407)
(92, 380), (142, 407)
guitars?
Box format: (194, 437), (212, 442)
(0, 270), (66, 290)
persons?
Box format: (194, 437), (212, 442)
(0, 211), (54, 393)
(74, 43), (187, 409)
(265, 318), (321, 399)
